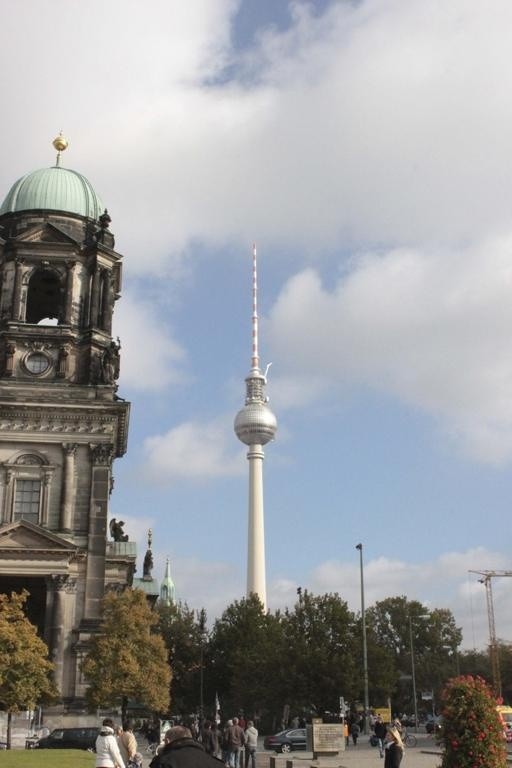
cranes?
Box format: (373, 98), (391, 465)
(468, 566), (512, 703)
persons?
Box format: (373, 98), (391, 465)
(347, 711), (387, 758)
(149, 726), (225, 767)
(110, 518), (128, 542)
(394, 713), (438, 734)
(95, 719), (161, 767)
(101, 337), (121, 385)
(185, 715), (258, 768)
(95, 208), (111, 243)
(275, 716), (306, 734)
(384, 728), (405, 768)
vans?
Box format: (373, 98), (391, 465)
(22, 726), (103, 752)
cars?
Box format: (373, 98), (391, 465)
(0, 741), (12, 749)
(400, 713), (445, 733)
(262, 727), (308, 754)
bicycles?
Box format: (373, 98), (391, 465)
(383, 728), (419, 748)
(145, 745), (156, 757)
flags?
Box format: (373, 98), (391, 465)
(216, 694), (221, 725)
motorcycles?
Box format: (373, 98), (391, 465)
(369, 735), (386, 747)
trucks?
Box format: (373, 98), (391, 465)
(495, 704), (512, 742)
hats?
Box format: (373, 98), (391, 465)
(386, 725), (400, 744)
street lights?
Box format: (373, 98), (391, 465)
(354, 541), (372, 734)
(294, 585), (306, 609)
(197, 618), (209, 717)
(409, 613), (434, 734)
